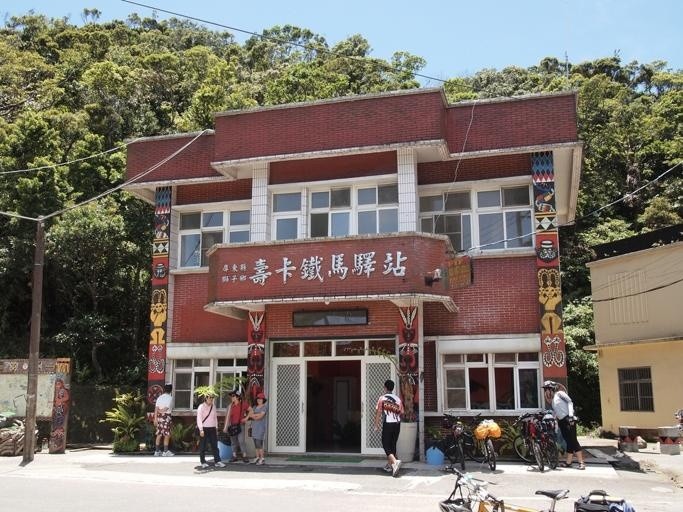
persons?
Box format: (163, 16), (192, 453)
(541, 380), (585, 469)
(373, 379), (404, 476)
(248, 392), (267, 466)
(222, 390), (253, 463)
(195, 393), (227, 468)
(153, 383), (176, 457)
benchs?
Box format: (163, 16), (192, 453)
(617, 424), (680, 453)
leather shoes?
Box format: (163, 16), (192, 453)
(228, 456), (238, 462)
(242, 457), (249, 463)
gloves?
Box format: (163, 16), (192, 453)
(568, 419), (576, 432)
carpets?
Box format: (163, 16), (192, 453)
(284, 455), (363, 464)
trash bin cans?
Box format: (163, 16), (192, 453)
(216, 433), (233, 460)
(425, 439), (445, 465)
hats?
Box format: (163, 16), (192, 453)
(165, 383), (172, 389)
(254, 393), (267, 404)
(229, 390), (242, 401)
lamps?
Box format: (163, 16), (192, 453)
(425, 268), (444, 283)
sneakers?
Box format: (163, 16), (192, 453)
(162, 450), (176, 457)
(250, 457), (265, 466)
(202, 463), (209, 469)
(382, 463), (393, 473)
(153, 450), (162, 457)
(392, 460), (402, 477)
(576, 463), (585, 470)
(557, 461), (572, 467)
(214, 462), (226, 468)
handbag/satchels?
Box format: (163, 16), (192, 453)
(228, 425), (242, 436)
(194, 425), (201, 436)
(248, 428), (252, 437)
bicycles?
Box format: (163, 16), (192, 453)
(438, 409), (562, 476)
(434, 465), (574, 512)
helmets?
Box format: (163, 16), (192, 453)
(540, 381), (559, 391)
